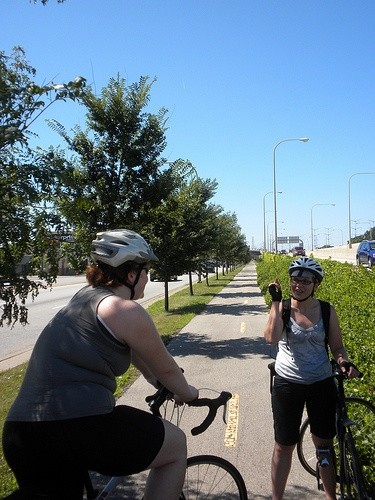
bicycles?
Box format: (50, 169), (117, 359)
(297, 357), (375, 500)
(53, 367), (247, 500)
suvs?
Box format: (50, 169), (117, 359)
(355, 240), (375, 269)
(291, 246), (306, 257)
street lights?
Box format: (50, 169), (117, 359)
(274, 137), (308, 254)
(311, 203), (336, 251)
(264, 191), (282, 250)
(266, 220), (284, 252)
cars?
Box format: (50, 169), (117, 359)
(279, 249), (287, 254)
(150, 270), (177, 282)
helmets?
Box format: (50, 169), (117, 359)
(288, 257), (323, 283)
(90, 228), (160, 268)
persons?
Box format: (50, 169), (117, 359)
(2, 229), (199, 500)
(264, 256), (360, 500)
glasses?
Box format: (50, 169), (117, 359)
(290, 277), (315, 285)
(134, 267), (150, 274)
